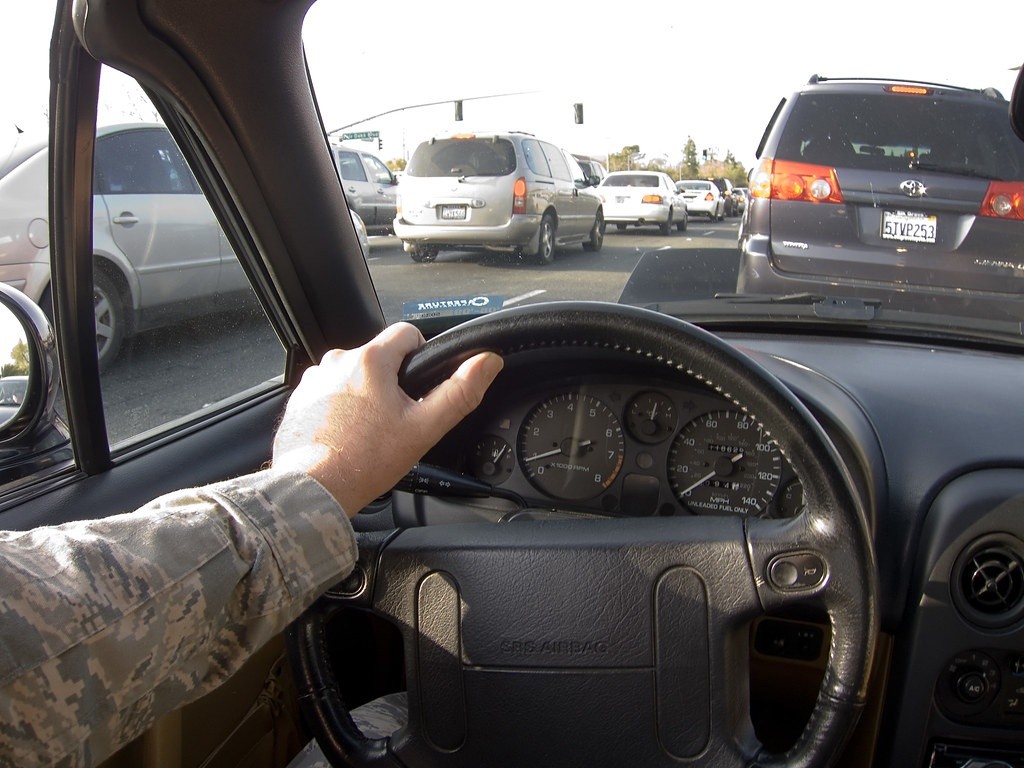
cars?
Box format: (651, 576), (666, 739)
(0, 376), (28, 425)
(675, 178), (749, 222)
(0, 121), (369, 376)
(597, 171), (688, 234)
(330, 144), (398, 236)
(578, 160), (607, 188)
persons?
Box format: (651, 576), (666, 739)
(0, 322), (504, 768)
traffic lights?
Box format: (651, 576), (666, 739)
(379, 138), (383, 149)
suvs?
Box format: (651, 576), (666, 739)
(393, 130), (606, 264)
(736, 74), (1023, 321)
